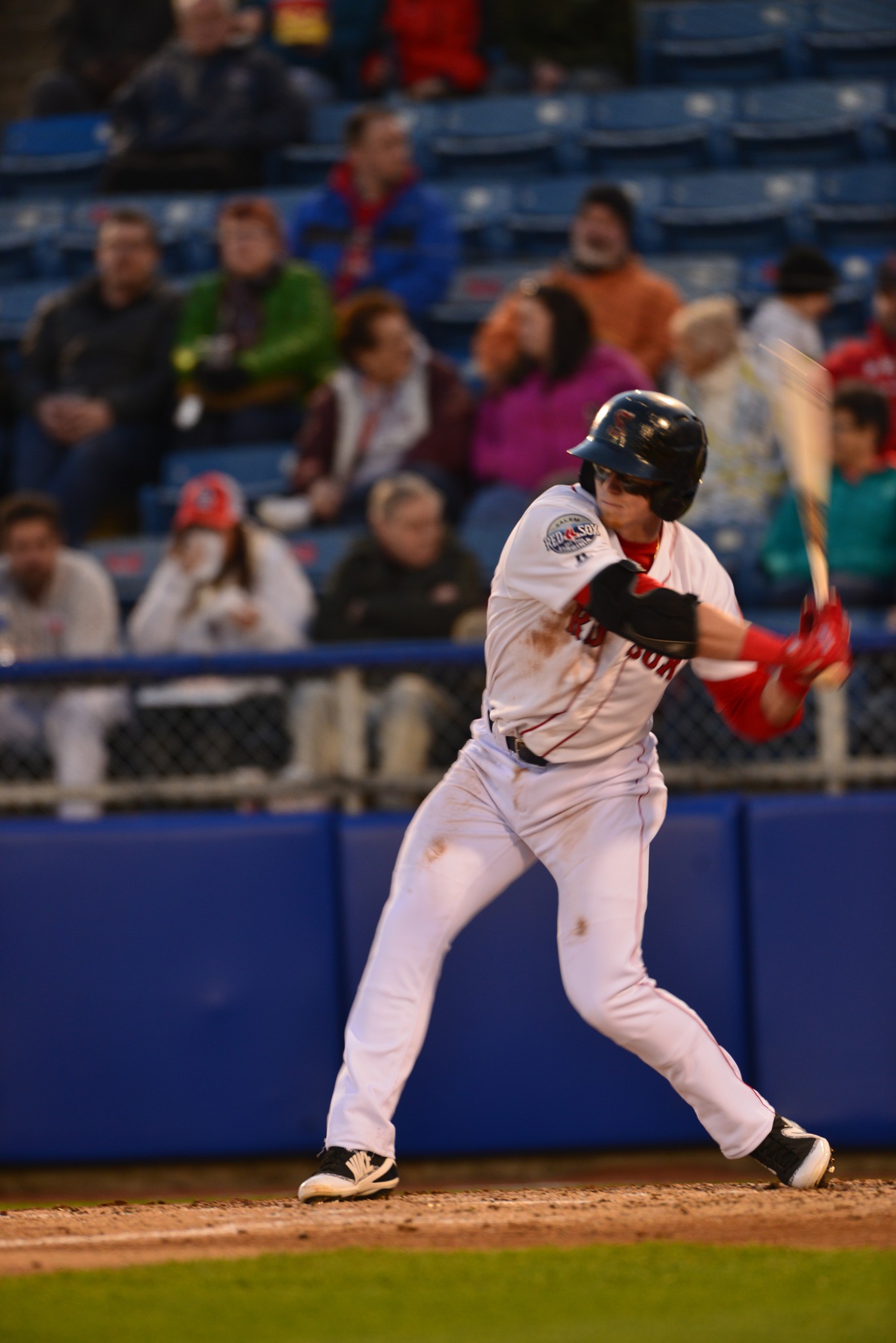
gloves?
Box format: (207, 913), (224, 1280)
(741, 584), (854, 703)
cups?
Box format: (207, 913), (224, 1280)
(186, 535), (226, 581)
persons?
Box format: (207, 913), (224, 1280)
(299, 389), (849, 1201)
(28, 0), (643, 198)
(289, 104), (459, 328)
(295, 295), (468, 524)
(474, 186), (681, 382)
(462, 286), (651, 584)
(306, 474), (488, 810)
(661, 247), (896, 630)
(168, 196), (332, 500)
(0, 494), (130, 820)
(21, 211), (183, 544)
(128, 474), (314, 812)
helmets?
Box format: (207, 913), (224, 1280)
(567, 393), (708, 522)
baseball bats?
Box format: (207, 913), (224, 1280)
(767, 346), (852, 686)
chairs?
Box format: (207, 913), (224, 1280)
(0, 0), (896, 714)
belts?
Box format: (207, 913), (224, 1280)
(490, 712), (550, 766)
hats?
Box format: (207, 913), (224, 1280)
(176, 476), (242, 532)
(582, 183), (632, 239)
(775, 245), (843, 294)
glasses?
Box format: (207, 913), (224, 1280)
(593, 462), (653, 496)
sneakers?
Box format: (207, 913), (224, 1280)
(746, 1114), (836, 1190)
(297, 1148), (398, 1205)
